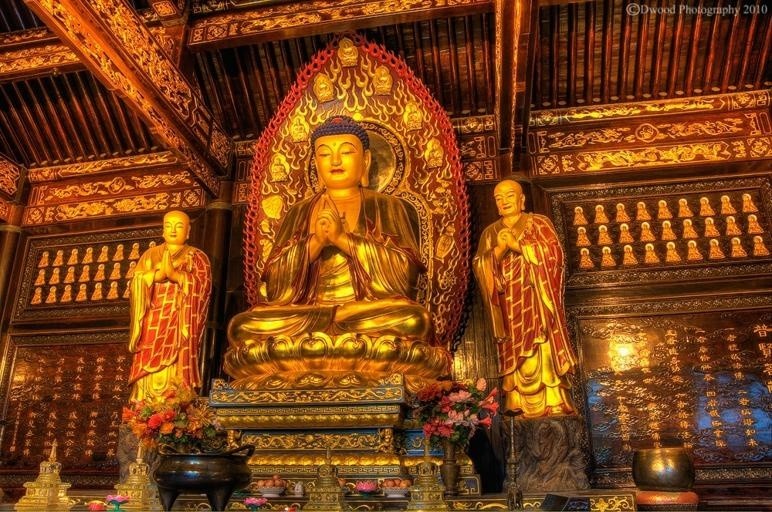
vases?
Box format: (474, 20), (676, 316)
(439, 435), (463, 499)
(151, 442), (256, 512)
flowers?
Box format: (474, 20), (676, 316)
(122, 377), (242, 455)
(404, 372), (504, 449)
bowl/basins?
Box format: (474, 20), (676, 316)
(381, 486), (411, 498)
(259, 486), (285, 498)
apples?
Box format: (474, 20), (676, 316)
(383, 478), (412, 488)
(258, 474), (288, 487)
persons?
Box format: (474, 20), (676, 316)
(30, 240), (156, 304)
(223, 115), (453, 382)
(573, 193), (770, 268)
(472, 179), (580, 420)
(127, 210), (213, 411)
